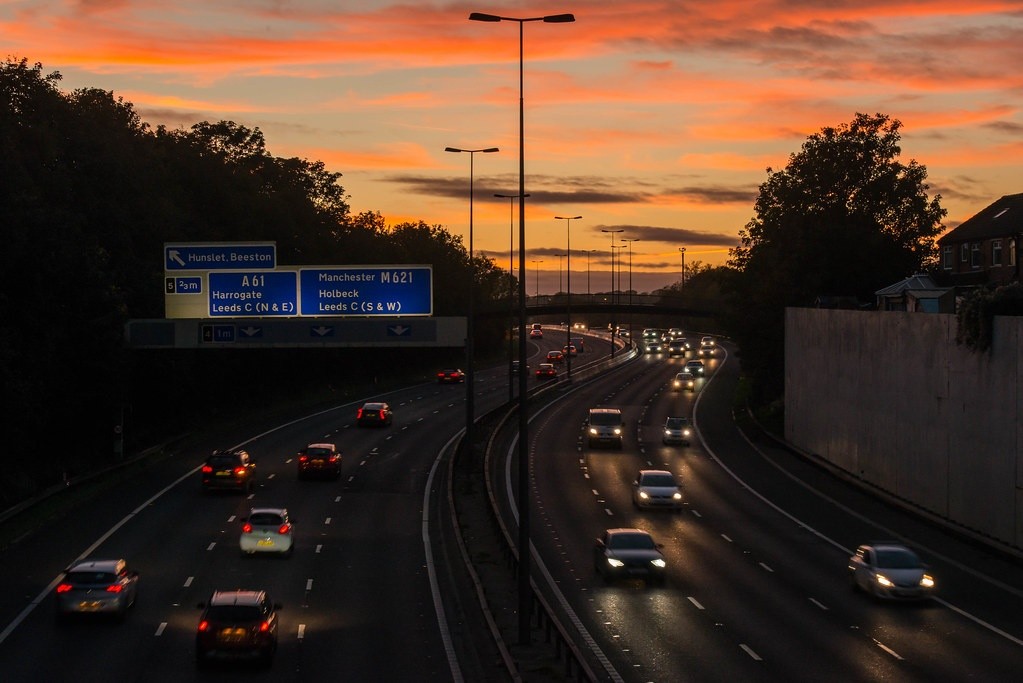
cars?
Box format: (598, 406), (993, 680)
(593, 529), (667, 584)
(661, 416), (691, 448)
(57, 558), (139, 614)
(632, 469), (683, 512)
(297, 443), (345, 479)
(240, 508), (296, 555)
(437, 323), (718, 394)
(357, 402), (393, 428)
(849, 544), (937, 603)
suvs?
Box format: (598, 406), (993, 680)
(195, 589), (283, 663)
(588, 408), (623, 448)
(202, 448), (256, 492)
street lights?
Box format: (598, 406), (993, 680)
(469, 11), (577, 648)
(621, 239), (640, 350)
(602, 230), (624, 361)
(611, 245), (628, 338)
(532, 260), (543, 307)
(553, 215), (582, 379)
(444, 146), (500, 261)
(555, 254), (567, 294)
(494, 194), (532, 400)
(582, 249), (596, 332)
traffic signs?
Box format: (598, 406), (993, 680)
(163, 245), (278, 271)
